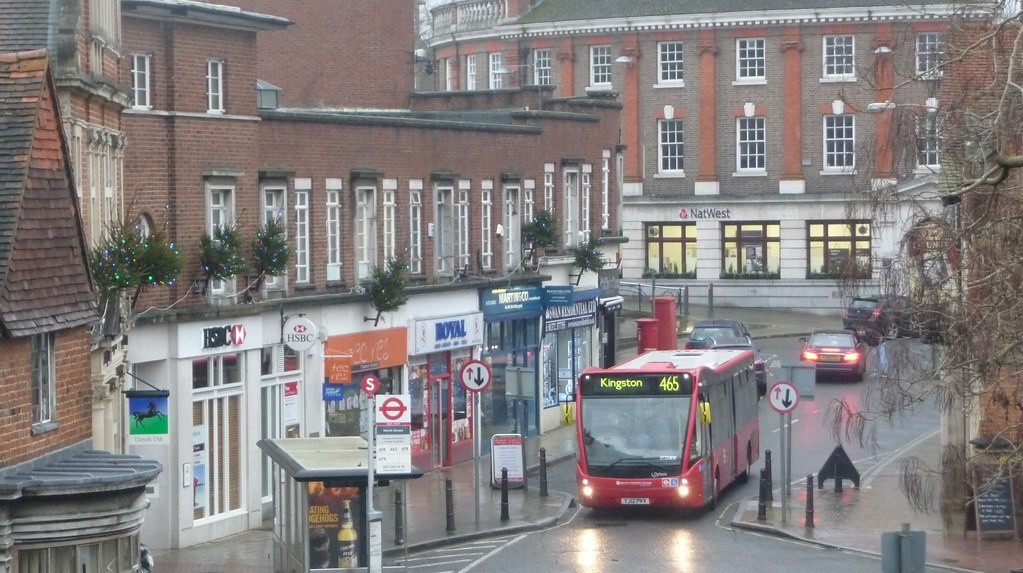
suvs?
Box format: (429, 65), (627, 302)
(843, 293), (948, 341)
(684, 319), (751, 349)
(701, 333), (767, 395)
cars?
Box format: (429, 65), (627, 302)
(799, 329), (867, 382)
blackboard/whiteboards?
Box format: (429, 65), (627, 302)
(973, 463), (1014, 531)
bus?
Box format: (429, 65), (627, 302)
(561, 348), (762, 513)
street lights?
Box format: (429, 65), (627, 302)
(941, 194), (969, 466)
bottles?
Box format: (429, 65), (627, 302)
(337, 500), (357, 568)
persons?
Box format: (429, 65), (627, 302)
(605, 408), (622, 425)
(310, 528), (330, 569)
